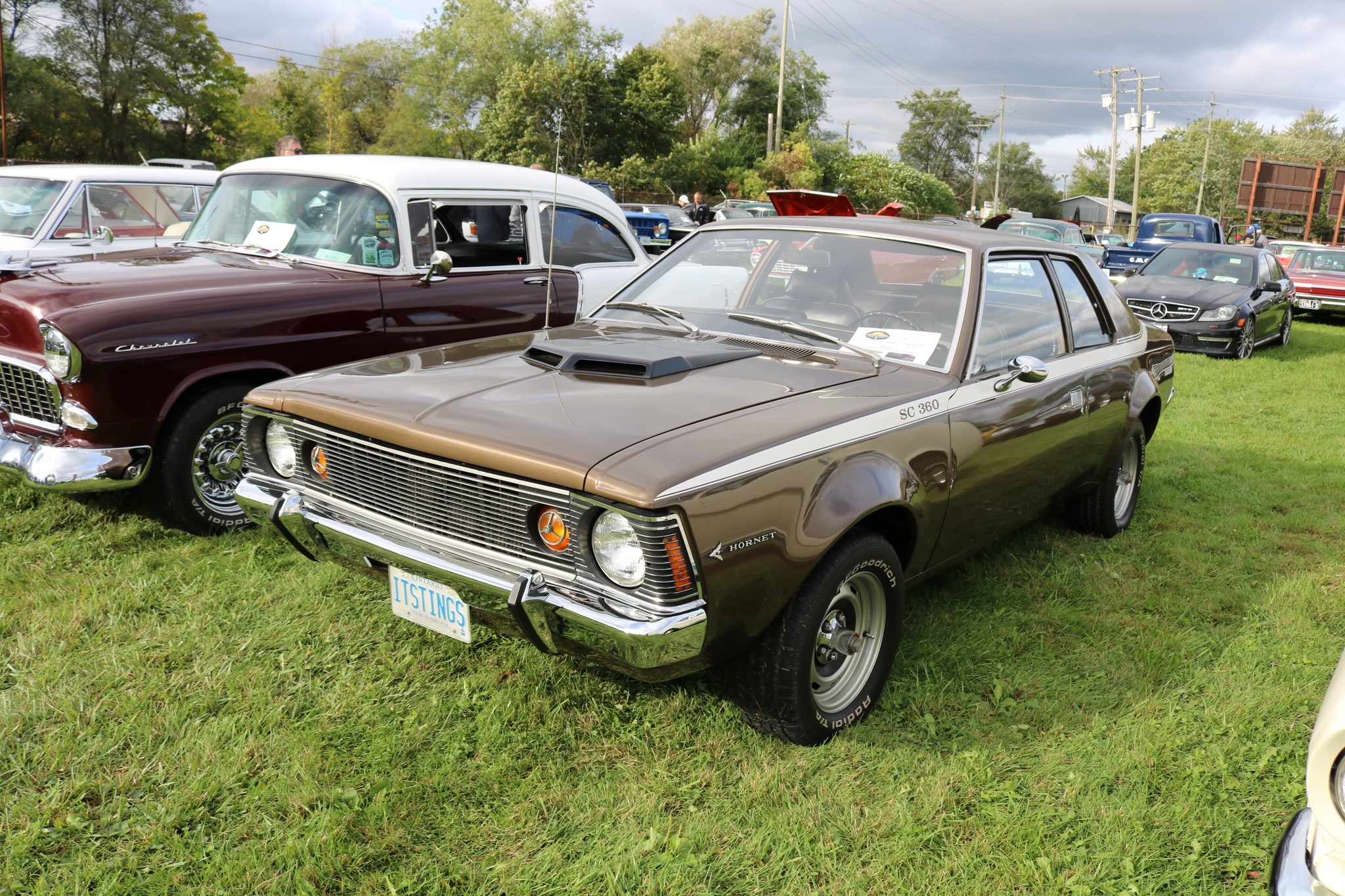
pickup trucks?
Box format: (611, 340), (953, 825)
(1099, 213), (1256, 281)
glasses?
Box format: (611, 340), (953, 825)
(284, 148), (304, 155)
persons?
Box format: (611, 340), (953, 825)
(470, 163), (562, 247)
(1246, 223), (1270, 250)
(1312, 238), (1319, 243)
(688, 192), (710, 226)
(274, 135), (303, 157)
(670, 195), (690, 209)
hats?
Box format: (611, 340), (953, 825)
(679, 195), (690, 203)
(1234, 235), (1241, 241)
(1243, 234), (1254, 242)
(1246, 223), (1261, 233)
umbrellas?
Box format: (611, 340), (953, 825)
(1072, 205), (1080, 228)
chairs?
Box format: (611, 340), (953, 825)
(762, 268), (864, 328)
(883, 281), (1008, 368)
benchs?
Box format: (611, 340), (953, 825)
(862, 290), (1124, 357)
(438, 241), (566, 267)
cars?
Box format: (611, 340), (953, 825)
(0, 152), (752, 543)
(234, 216), (1177, 748)
(714, 189), (1131, 285)
(1265, 241), (1345, 312)
(1114, 241), (1296, 361)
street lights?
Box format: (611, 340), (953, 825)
(976, 152), (1000, 218)
(1186, 160), (1205, 215)
